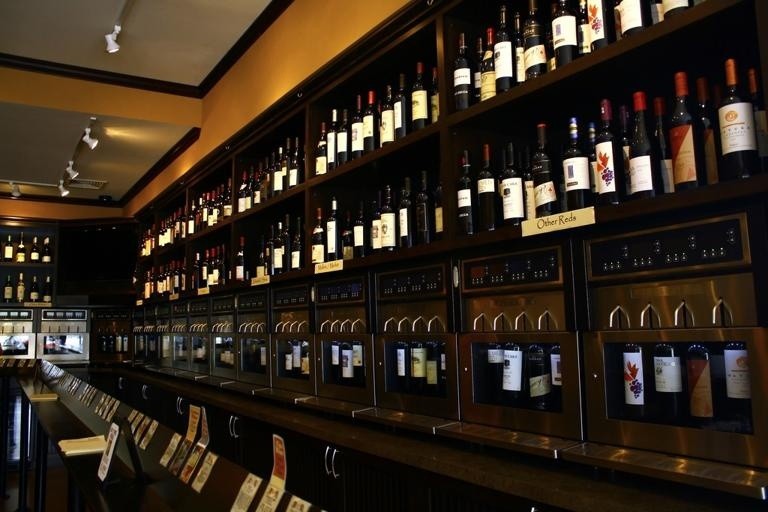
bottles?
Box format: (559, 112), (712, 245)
(441, 342), (448, 399)
(31, 237), (40, 262)
(43, 275), (51, 302)
(395, 342), (410, 395)
(620, 0), (652, 36)
(712, 85), (723, 109)
(434, 171), (443, 239)
(411, 343), (427, 396)
(97, 328), (128, 353)
(613, 0), (622, 41)
(236, 236), (247, 281)
(481, 27), (496, 102)
(551, 0), (578, 69)
(265, 225), (274, 276)
(341, 343), (354, 378)
(348, 95), (362, 161)
(516, 12), (525, 85)
(456, 148), (476, 234)
(380, 85), (394, 145)
(477, 144), (501, 229)
(399, 177), (412, 246)
(274, 222), (282, 273)
(3, 275), (13, 302)
(629, 92), (660, 199)
(594, 100), (626, 205)
(563, 116), (591, 210)
(453, 33), (472, 111)
(686, 345), (716, 426)
(314, 123), (326, 177)
(30, 276), (39, 301)
(42, 236), (51, 263)
(652, 344), (683, 424)
(533, 124), (559, 218)
(587, 0), (616, 50)
(694, 78), (718, 186)
(648, 0), (663, 25)
(427, 344), (441, 398)
(668, 72), (705, 191)
(662, 0), (692, 21)
(415, 171), (430, 245)
(362, 90), (377, 155)
(326, 196), (341, 261)
(488, 344), (502, 403)
(473, 37), (482, 105)
(577, 0), (591, 55)
(301, 340), (310, 379)
(747, 67), (768, 176)
(353, 341), (363, 367)
(502, 141), (525, 225)
(237, 147), (282, 212)
(527, 344), (550, 409)
(588, 123), (596, 195)
(17, 232), (25, 263)
(653, 96), (675, 194)
(285, 341), (293, 373)
(282, 138), (288, 190)
(393, 74), (409, 140)
(16, 273), (25, 302)
(381, 184), (396, 249)
(331, 341), (339, 366)
(623, 345), (644, 418)
(411, 62), (427, 132)
(428, 62), (439, 124)
(620, 105), (631, 199)
(257, 252), (265, 277)
(341, 211), (354, 259)
(723, 343), (751, 433)
(718, 58), (758, 181)
(548, 346), (563, 411)
(282, 214), (292, 271)
(292, 217), (303, 270)
(522, 145), (535, 219)
(225, 342), (231, 364)
(257, 341), (267, 373)
(327, 109), (336, 172)
(494, 5), (517, 94)
(373, 191), (381, 251)
(5, 234), (12, 261)
(354, 202), (367, 257)
(136, 178), (234, 301)
(524, 0), (548, 79)
(502, 344), (524, 406)
(289, 137), (303, 188)
(312, 208), (325, 265)
(336, 108), (347, 166)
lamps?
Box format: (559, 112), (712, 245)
(66, 161), (79, 180)
(57, 179), (70, 197)
(82, 127), (99, 152)
(9, 183), (22, 197)
(104, 25), (121, 54)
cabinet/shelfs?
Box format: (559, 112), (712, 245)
(0, 221), (59, 307)
(111, 367), (768, 511)
(108, 0), (768, 500)
